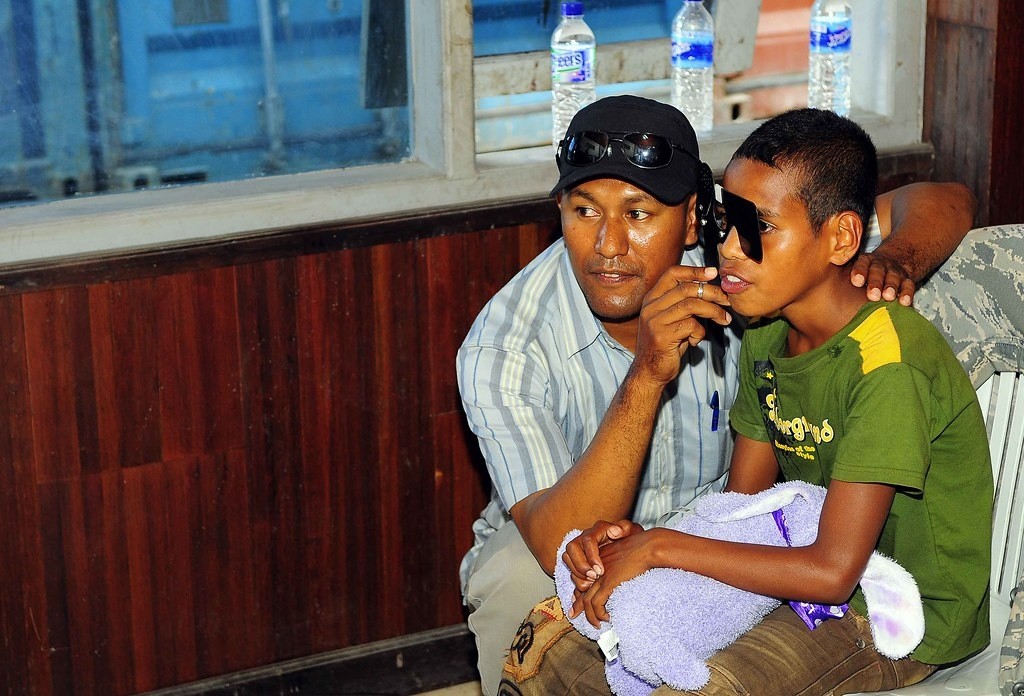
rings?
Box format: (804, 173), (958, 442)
(697, 282), (704, 298)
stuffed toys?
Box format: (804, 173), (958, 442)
(554, 479), (927, 694)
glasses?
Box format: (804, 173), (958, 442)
(555, 129), (701, 170)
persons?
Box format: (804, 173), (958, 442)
(562, 106), (993, 694)
(456, 97), (976, 695)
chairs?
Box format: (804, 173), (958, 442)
(844, 225), (1024, 696)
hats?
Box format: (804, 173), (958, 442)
(549, 95), (699, 207)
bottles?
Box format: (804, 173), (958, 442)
(670, 0), (715, 140)
(549, 2), (595, 155)
(808, 0), (853, 120)
